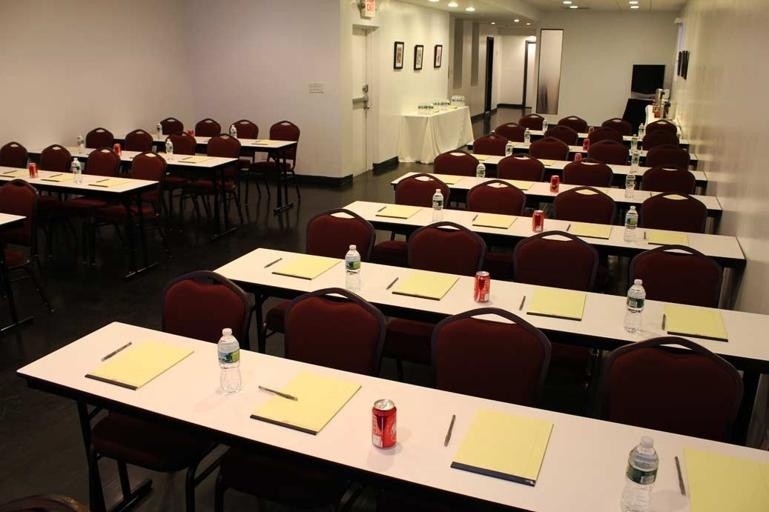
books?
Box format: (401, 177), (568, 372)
(685, 446), (767, 512)
(250, 370), (361, 437)
(450, 406), (554, 487)
(392, 270), (460, 300)
(668, 303), (728, 344)
(525, 287), (589, 321)
(0, 137), (276, 188)
(273, 254), (338, 278)
(85, 340), (195, 389)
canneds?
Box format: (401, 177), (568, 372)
(186, 128), (194, 138)
(550, 174), (560, 194)
(588, 125), (594, 134)
(583, 139), (590, 152)
(473, 270), (491, 304)
(28, 162), (39, 178)
(113, 143), (121, 157)
(370, 396), (398, 451)
(575, 152), (581, 162)
(531, 210), (544, 233)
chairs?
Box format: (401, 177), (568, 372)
(588, 336), (746, 445)
(86, 269), (252, 512)
(330, 112), (747, 309)
(0, 492), (90, 512)
(429, 307), (553, 408)
(213, 287), (389, 512)
(0, 115), (302, 281)
(374, 220), (487, 381)
(258, 207), (377, 358)
(509, 229), (599, 420)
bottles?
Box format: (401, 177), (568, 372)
(624, 122), (646, 199)
(418, 95), (466, 115)
(474, 117), (549, 183)
(623, 205), (639, 241)
(154, 121), (177, 164)
(69, 131), (85, 191)
(345, 244), (362, 292)
(230, 123), (238, 140)
(431, 187), (444, 221)
(216, 326), (242, 395)
(621, 277), (646, 334)
(620, 433), (659, 512)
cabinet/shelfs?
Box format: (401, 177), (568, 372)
(622, 97), (655, 135)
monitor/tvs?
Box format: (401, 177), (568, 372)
(630, 64), (666, 99)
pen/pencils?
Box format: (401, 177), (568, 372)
(96, 179), (109, 182)
(472, 215), (478, 221)
(675, 455), (686, 495)
(50, 174), (63, 178)
(662, 314), (666, 330)
(386, 277), (399, 289)
(258, 385), (297, 401)
(3, 170), (17, 174)
(519, 296), (526, 310)
(377, 207), (386, 212)
(444, 414), (456, 447)
(644, 232), (646, 239)
(103, 342), (132, 360)
(264, 258), (282, 268)
(566, 224), (571, 231)
(183, 157), (192, 160)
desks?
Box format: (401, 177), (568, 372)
(397, 104), (475, 165)
(0, 174), (56, 338)
(212, 246), (768, 408)
(14, 320), (769, 512)
(643, 104), (682, 139)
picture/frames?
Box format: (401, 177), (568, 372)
(393, 41), (404, 69)
(414, 44), (424, 70)
(433, 44), (443, 68)
